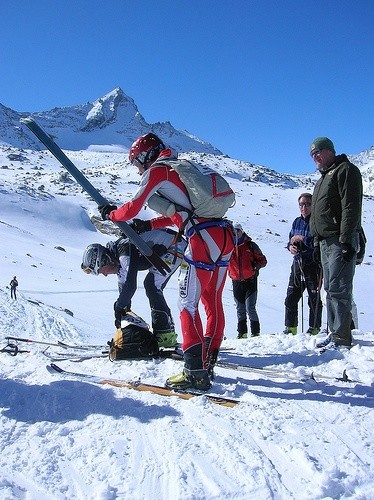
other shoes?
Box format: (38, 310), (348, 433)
(316, 336), (350, 355)
(166, 371), (214, 392)
(154, 332), (177, 347)
(283, 327), (297, 335)
(237, 334), (247, 339)
(307, 327), (320, 334)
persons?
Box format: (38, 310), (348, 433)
(98, 132), (236, 390)
(81, 227), (189, 359)
(10, 276), (18, 300)
(282, 193), (324, 336)
(228, 223), (267, 339)
(310, 136), (363, 354)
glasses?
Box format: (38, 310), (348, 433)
(81, 263), (93, 274)
(299, 202), (312, 207)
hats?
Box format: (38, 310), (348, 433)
(310, 137), (336, 154)
(233, 222), (243, 231)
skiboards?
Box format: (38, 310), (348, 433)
(40, 339), (249, 362)
(45, 362), (241, 409)
(20, 115), (173, 277)
(162, 345), (362, 388)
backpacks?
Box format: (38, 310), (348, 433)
(355, 227), (367, 265)
(150, 158), (235, 219)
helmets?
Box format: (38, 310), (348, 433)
(83, 243), (104, 275)
(129, 133), (161, 162)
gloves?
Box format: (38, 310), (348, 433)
(124, 302), (133, 313)
(98, 204), (119, 221)
(114, 304), (126, 328)
(119, 218), (152, 240)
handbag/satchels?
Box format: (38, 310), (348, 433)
(107, 324), (160, 361)
